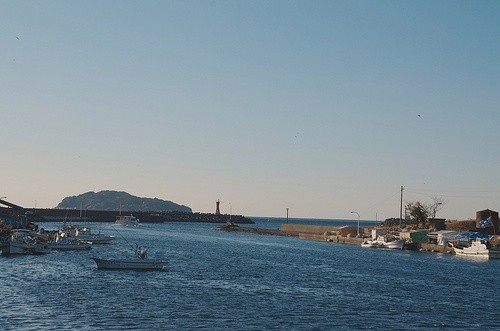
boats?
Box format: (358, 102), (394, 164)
(374, 235), (404, 250)
(448, 239), (489, 255)
(89, 244), (169, 272)
(1, 214), (114, 254)
(110, 216), (145, 229)
(360, 239), (378, 247)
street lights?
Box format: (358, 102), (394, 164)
(351, 211), (360, 235)
(429, 203), (442, 219)
